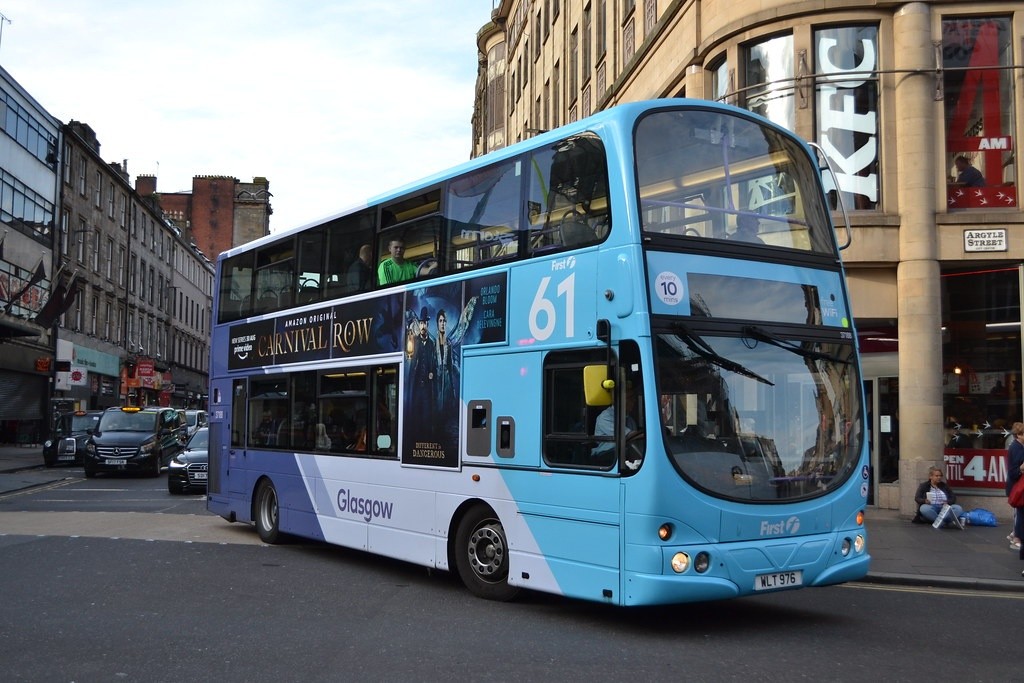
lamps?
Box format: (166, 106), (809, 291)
(123, 356), (138, 378)
(188, 390), (193, 398)
(195, 392), (203, 400)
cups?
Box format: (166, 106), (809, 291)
(960, 517), (965, 526)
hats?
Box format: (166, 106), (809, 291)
(417, 306), (430, 322)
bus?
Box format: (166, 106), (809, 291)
(204, 97), (873, 608)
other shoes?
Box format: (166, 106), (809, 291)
(943, 521), (957, 528)
(1006, 532), (1020, 551)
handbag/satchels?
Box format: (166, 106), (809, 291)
(1007, 473), (1024, 508)
(958, 508), (998, 526)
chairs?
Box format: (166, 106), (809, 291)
(239, 259), (389, 319)
(275, 415), (305, 447)
(559, 210), (599, 246)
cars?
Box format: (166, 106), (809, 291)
(43, 410), (105, 468)
(167, 427), (208, 494)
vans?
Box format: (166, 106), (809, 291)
(84, 404), (187, 477)
(185, 409), (206, 438)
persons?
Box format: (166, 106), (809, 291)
(257, 409), (279, 446)
(432, 309), (456, 420)
(592, 393), (637, 456)
(346, 244), (374, 293)
(991, 381), (1005, 401)
(378, 237), (438, 287)
(1004, 422), (1024, 550)
(954, 156), (987, 187)
(912, 465), (962, 529)
(886, 410), (899, 477)
(409, 307), (438, 432)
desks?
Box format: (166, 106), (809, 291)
(957, 428), (1011, 448)
